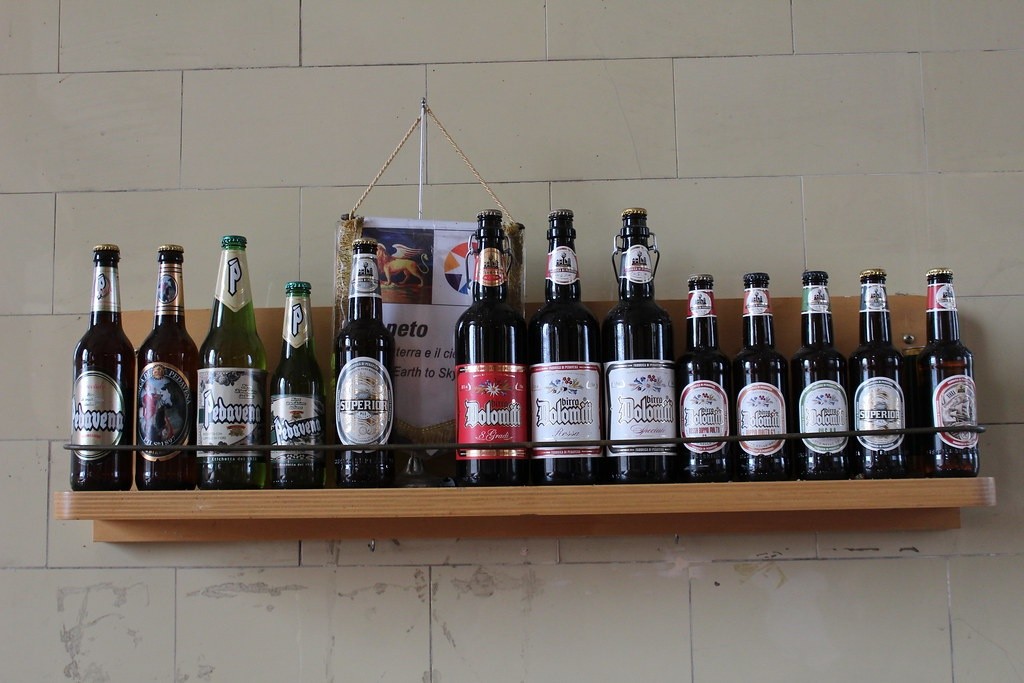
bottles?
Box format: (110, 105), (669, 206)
(526, 208), (604, 484)
(136, 243), (198, 491)
(69, 243), (135, 491)
(916, 266), (980, 479)
(452, 209), (529, 487)
(677, 274), (731, 481)
(603, 208), (682, 483)
(197, 233), (270, 489)
(269, 280), (327, 490)
(730, 272), (789, 481)
(332, 237), (395, 488)
(790, 267), (848, 480)
(848, 268), (912, 477)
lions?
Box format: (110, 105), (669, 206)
(377, 245), (429, 290)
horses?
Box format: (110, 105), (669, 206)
(139, 390), (173, 441)
